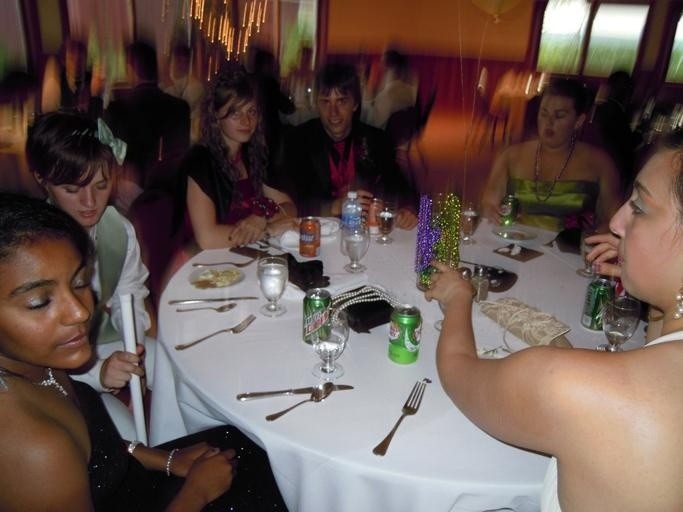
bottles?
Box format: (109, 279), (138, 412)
(458, 267), (488, 303)
(339, 192), (362, 256)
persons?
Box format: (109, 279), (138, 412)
(1, 21), (419, 511)
(423, 126), (683, 512)
(478, 76), (621, 242)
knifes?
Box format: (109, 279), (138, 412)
(166, 296), (258, 304)
(233, 384), (356, 403)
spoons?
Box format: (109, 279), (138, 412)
(262, 383), (333, 423)
(174, 302), (236, 312)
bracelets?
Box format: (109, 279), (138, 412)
(648, 312), (664, 322)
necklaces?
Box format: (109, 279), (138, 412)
(533, 136), (576, 202)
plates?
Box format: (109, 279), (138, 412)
(188, 265), (246, 288)
(299, 212), (340, 238)
(490, 226), (535, 240)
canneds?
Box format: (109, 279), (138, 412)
(580, 278), (614, 332)
(615, 277), (627, 298)
(367, 198), (385, 226)
(498, 194), (518, 227)
(299, 216), (321, 258)
(301, 287), (333, 346)
(387, 303), (423, 365)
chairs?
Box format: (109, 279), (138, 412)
(410, 83), (440, 174)
(382, 107), (418, 190)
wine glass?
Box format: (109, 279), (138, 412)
(592, 294), (642, 350)
(573, 228), (598, 279)
(258, 255), (289, 318)
(373, 200), (398, 246)
(308, 307), (348, 381)
(339, 223), (370, 272)
(458, 199), (479, 245)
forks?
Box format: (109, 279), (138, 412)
(190, 258), (259, 267)
(172, 312), (257, 351)
(371, 380), (426, 458)
